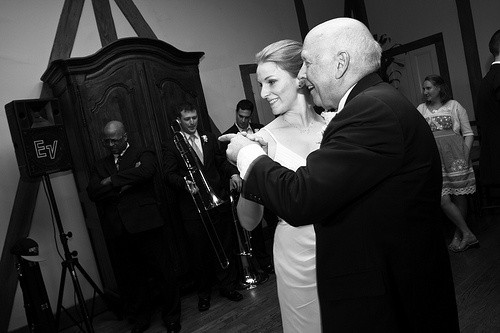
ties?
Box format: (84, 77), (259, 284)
(190, 136), (203, 164)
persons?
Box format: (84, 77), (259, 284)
(160, 103), (244, 311)
(219, 100), (278, 282)
(218, 17), (460, 333)
(237, 40), (337, 333)
(417, 74), (479, 252)
(86, 120), (182, 333)
(478, 30), (500, 184)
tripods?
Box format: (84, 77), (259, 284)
(45, 175), (112, 332)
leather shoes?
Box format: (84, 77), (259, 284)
(198, 292), (211, 310)
(131, 328), (145, 333)
(166, 320), (181, 333)
(219, 289), (242, 301)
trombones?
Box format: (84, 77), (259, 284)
(170, 118), (230, 270)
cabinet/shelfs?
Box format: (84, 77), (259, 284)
(40, 37), (245, 316)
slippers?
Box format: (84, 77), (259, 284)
(454, 235), (480, 252)
(448, 235), (462, 251)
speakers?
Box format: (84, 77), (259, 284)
(5, 99), (73, 177)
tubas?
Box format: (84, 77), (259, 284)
(230, 179), (267, 291)
